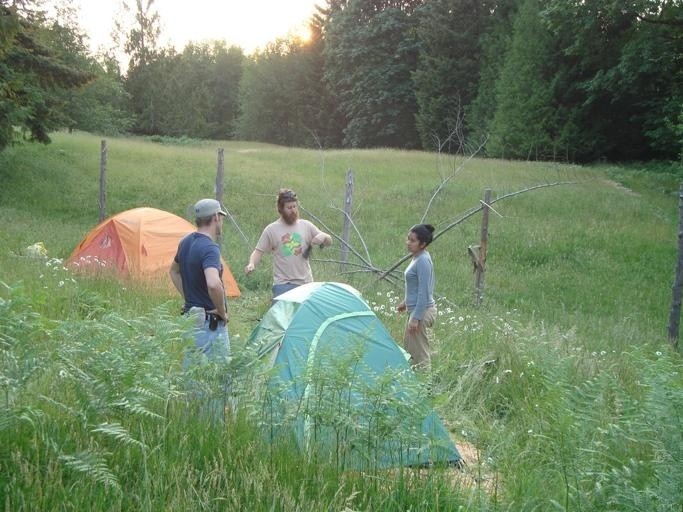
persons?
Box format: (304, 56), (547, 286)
(396, 223), (436, 378)
(245, 186), (333, 299)
(169, 197), (230, 363)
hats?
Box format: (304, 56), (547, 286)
(192, 198), (227, 218)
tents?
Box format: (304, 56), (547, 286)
(236, 278), (461, 470)
(65, 206), (241, 298)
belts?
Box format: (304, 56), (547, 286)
(183, 308), (224, 321)
